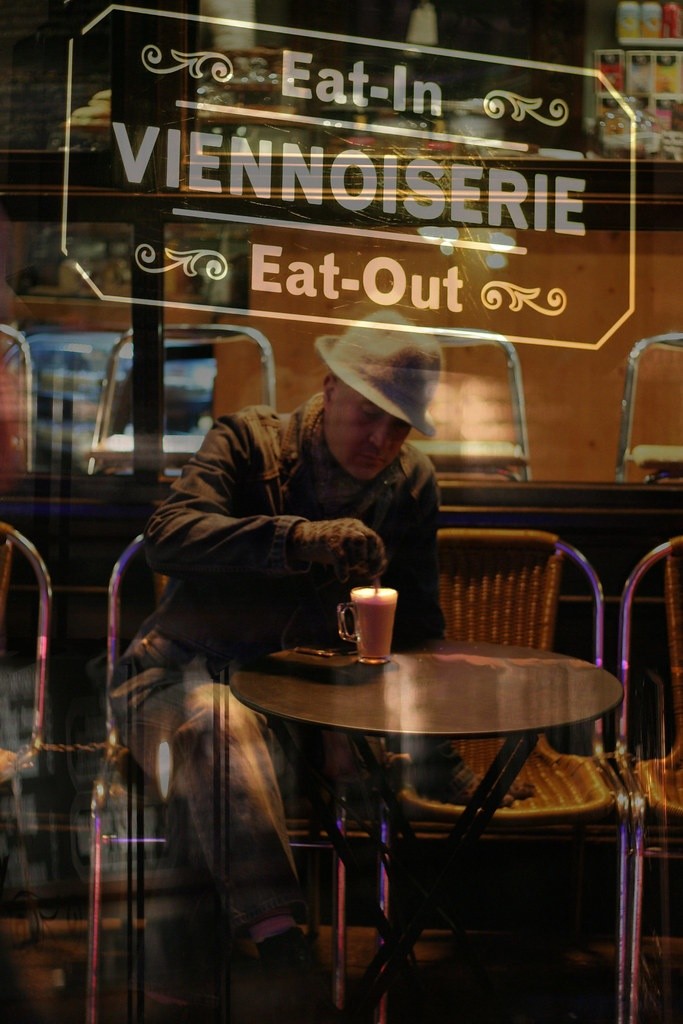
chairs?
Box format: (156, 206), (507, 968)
(338, 327), (539, 485)
(0, 315), (42, 481)
(375, 524), (611, 1024)
(612, 327), (683, 489)
(81, 533), (348, 1024)
(81, 318), (283, 478)
(0, 520), (62, 1024)
(617, 533), (683, 1024)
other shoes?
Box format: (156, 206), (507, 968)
(256, 926), (352, 1024)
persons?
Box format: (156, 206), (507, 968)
(107, 308), (535, 1024)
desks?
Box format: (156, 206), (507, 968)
(222, 638), (623, 1024)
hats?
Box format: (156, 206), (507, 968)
(315, 308), (441, 437)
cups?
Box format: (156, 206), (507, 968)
(336, 586), (398, 664)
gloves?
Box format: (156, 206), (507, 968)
(286, 518), (389, 584)
(453, 774), (537, 806)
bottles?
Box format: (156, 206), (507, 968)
(599, 0), (683, 160)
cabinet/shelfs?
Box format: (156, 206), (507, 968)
(1, 0), (682, 468)
